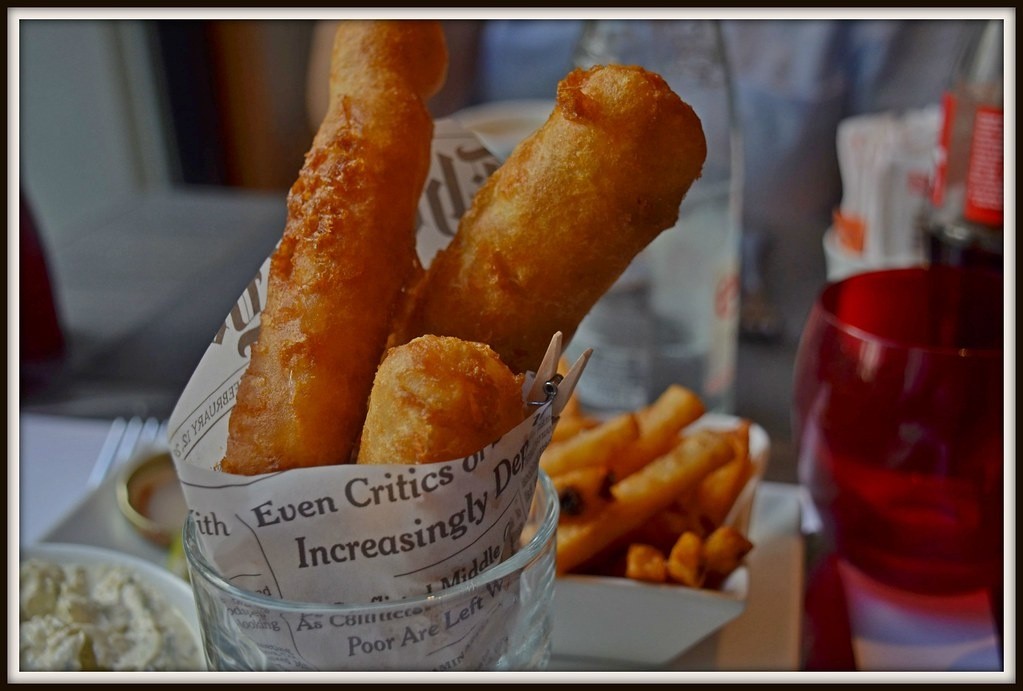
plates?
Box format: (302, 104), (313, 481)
(18, 538), (212, 674)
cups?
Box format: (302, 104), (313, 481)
(788, 267), (1003, 614)
(180, 470), (559, 671)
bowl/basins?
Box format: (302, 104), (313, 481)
(538, 413), (779, 663)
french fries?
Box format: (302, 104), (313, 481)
(523, 354), (754, 588)
